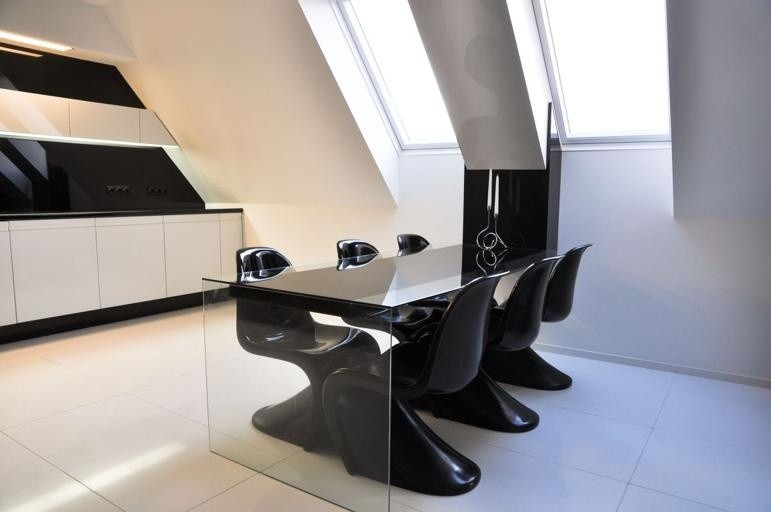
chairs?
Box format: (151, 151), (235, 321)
(323, 271), (540, 495)
(397, 236), (454, 319)
(417, 255), (573, 391)
(335, 242), (433, 342)
(237, 247), (382, 451)
(488, 243), (593, 341)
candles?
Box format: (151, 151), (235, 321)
(476, 167), (508, 277)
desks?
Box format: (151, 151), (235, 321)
(197, 239), (568, 510)
